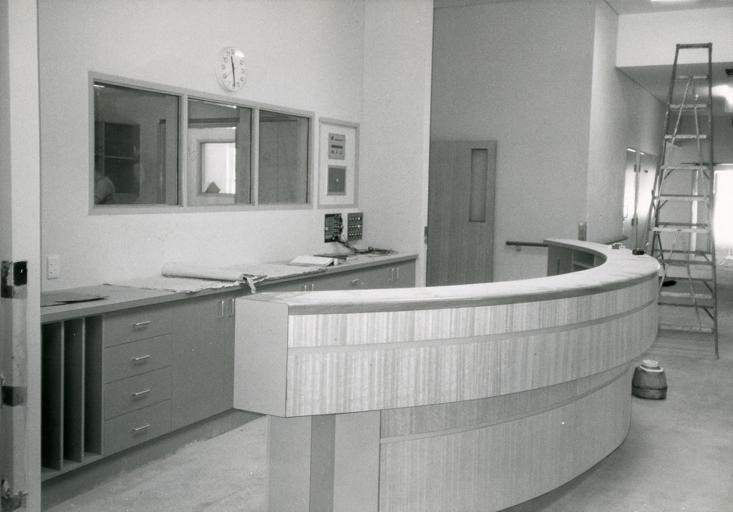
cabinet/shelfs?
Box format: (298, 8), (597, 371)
(172, 285), (236, 436)
(99, 284), (173, 463)
(256, 260), (429, 293)
(43, 288), (103, 482)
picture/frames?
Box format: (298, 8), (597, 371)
(318, 118), (361, 209)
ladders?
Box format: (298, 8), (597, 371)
(641, 41), (719, 359)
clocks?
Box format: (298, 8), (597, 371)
(214, 44), (248, 92)
